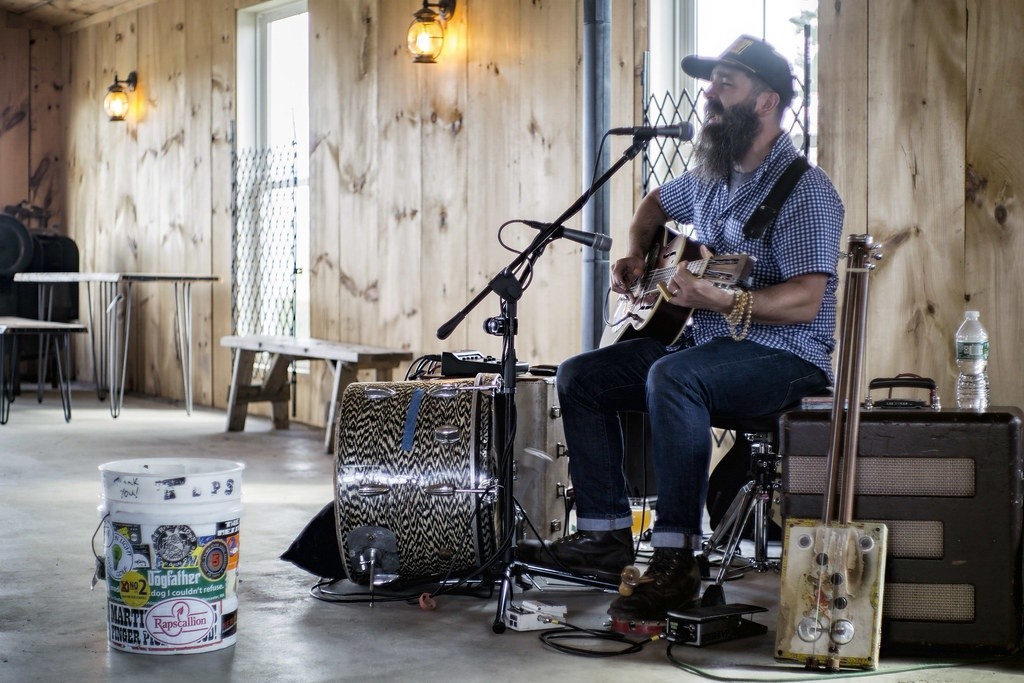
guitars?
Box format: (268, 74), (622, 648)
(597, 225), (758, 352)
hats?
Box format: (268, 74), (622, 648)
(681, 35), (793, 106)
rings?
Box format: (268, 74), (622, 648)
(616, 280), (624, 286)
(670, 287), (680, 296)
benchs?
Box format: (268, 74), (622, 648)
(0, 315), (89, 426)
(220, 334), (414, 455)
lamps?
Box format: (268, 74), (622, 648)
(103, 71), (137, 121)
(407, 0), (456, 64)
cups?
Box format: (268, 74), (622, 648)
(628, 498), (652, 536)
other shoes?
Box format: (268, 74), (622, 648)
(607, 548), (701, 620)
(518, 527), (634, 583)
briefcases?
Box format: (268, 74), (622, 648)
(779, 378), (1024, 656)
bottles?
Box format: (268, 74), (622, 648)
(956, 311), (991, 410)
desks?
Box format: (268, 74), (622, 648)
(13, 272), (222, 418)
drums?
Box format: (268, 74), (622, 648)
(335, 372), (512, 585)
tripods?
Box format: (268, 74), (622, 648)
(396, 142), (649, 635)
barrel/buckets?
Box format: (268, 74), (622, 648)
(91, 457), (247, 656)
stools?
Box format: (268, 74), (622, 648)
(695, 388), (835, 607)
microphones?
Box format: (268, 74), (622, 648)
(524, 220), (613, 252)
(609, 122), (696, 141)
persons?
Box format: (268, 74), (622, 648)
(517, 35), (845, 623)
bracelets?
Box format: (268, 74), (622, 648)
(723, 287), (754, 343)
